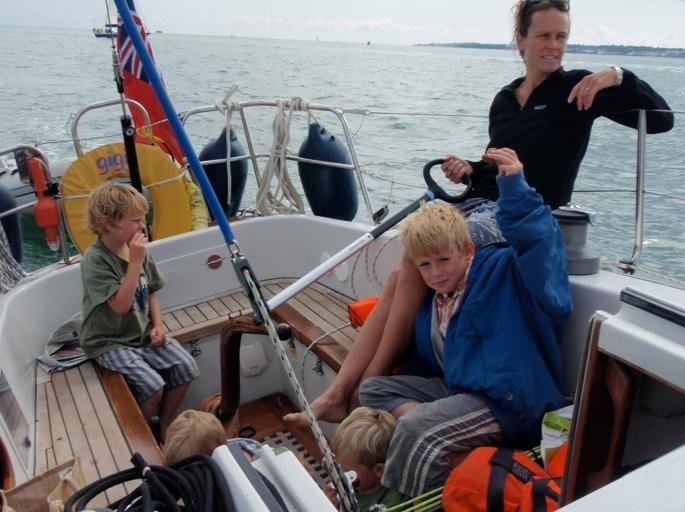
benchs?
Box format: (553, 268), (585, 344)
(151, 281), (404, 386)
(31, 358), (184, 512)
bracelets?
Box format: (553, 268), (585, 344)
(611, 64), (625, 88)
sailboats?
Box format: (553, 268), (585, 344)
(91, 0), (152, 39)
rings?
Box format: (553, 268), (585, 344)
(447, 170), (454, 177)
(586, 86), (591, 91)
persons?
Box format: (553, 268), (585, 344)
(77, 180), (200, 448)
(328, 404), (415, 511)
(276, 0), (680, 434)
(159, 406), (265, 508)
(356, 143), (579, 512)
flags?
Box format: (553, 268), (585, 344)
(115, 0), (187, 170)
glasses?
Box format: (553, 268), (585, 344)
(519, 0), (570, 24)
